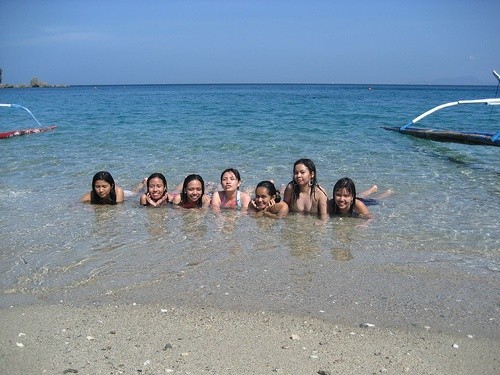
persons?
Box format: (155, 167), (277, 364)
(140, 172), (188, 206)
(283, 158), (329, 226)
(172, 173), (214, 221)
(328, 176), (400, 230)
(79, 170), (148, 212)
(248, 179), (289, 219)
(211, 168), (256, 228)
